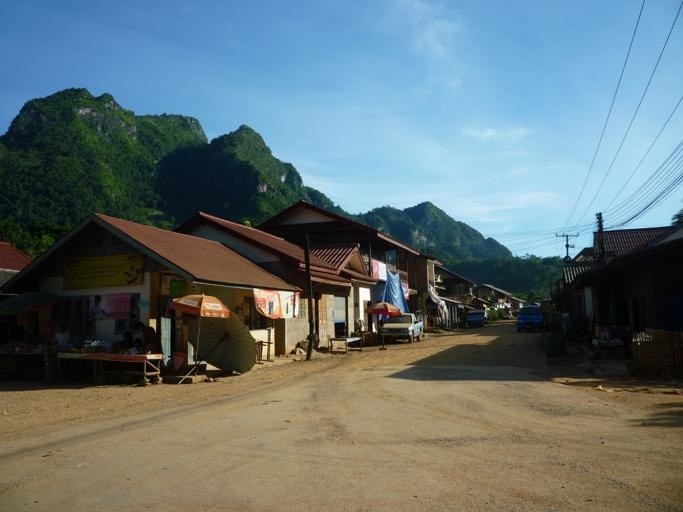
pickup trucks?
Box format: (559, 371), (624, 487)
(380, 313), (424, 344)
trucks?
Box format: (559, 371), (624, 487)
(515, 306), (544, 332)
(467, 309), (486, 329)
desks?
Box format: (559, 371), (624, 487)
(56, 350), (164, 385)
(329, 337), (363, 354)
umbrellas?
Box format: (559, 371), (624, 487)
(365, 301), (403, 350)
(178, 308), (258, 384)
(171, 292), (229, 376)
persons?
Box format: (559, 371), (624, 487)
(136, 321), (156, 348)
(90, 294), (107, 320)
